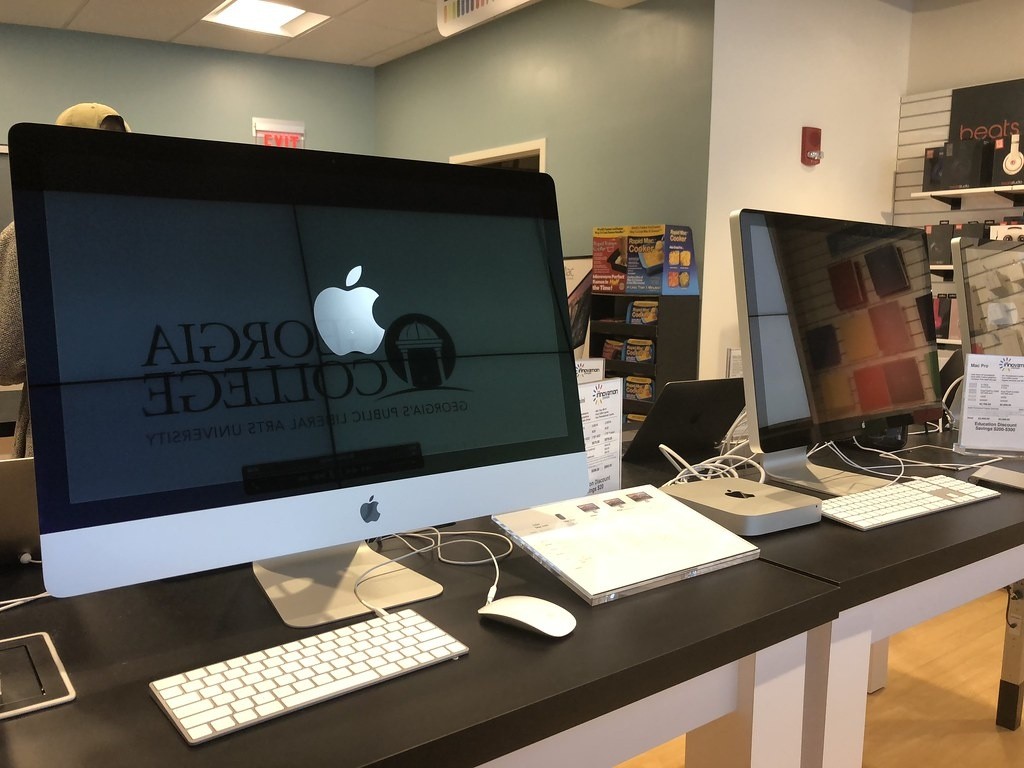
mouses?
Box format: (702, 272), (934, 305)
(476, 595), (576, 637)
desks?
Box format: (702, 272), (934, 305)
(620, 424), (1024, 768)
(0, 490), (840, 768)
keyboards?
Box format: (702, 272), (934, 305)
(821, 474), (1002, 531)
(149, 609), (468, 746)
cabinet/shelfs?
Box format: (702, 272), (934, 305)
(911, 183), (1024, 344)
(589, 292), (699, 415)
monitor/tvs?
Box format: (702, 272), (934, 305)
(949, 236), (1024, 363)
(729, 208), (945, 498)
(7, 123), (591, 630)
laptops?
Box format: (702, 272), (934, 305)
(621, 378), (745, 463)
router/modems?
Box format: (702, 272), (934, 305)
(658, 476), (823, 536)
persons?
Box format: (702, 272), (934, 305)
(0, 101), (133, 460)
